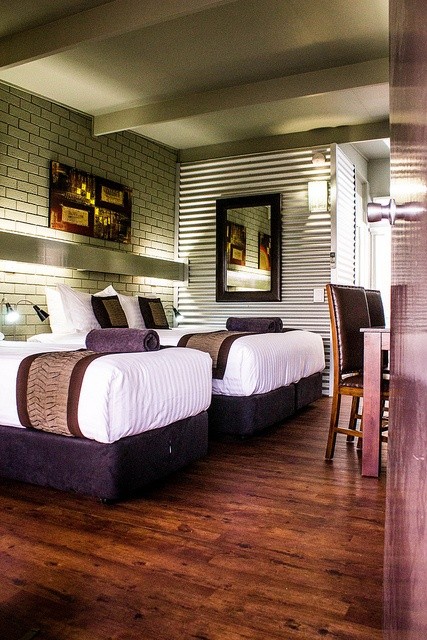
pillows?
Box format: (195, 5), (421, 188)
(55, 282), (116, 332)
(117, 294), (155, 329)
(91, 294), (129, 327)
(137, 294), (170, 328)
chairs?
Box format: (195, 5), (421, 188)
(324, 284), (389, 460)
(356, 288), (389, 449)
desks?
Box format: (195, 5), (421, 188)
(359, 326), (388, 478)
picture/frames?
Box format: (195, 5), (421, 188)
(227, 220), (246, 267)
(258, 231), (271, 270)
(48, 160), (132, 245)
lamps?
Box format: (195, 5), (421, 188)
(163, 304), (184, 322)
(15, 299), (50, 322)
(1, 297), (20, 323)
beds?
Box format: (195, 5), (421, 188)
(1, 335), (213, 505)
(28, 326), (324, 434)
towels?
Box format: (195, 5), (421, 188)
(226, 316), (275, 332)
(87, 329), (160, 352)
(248, 315), (284, 332)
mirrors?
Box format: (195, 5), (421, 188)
(213, 193), (282, 303)
